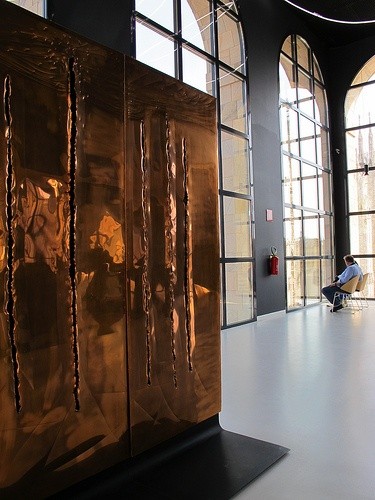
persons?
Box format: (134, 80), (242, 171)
(321, 255), (364, 312)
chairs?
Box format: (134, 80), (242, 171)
(340, 272), (369, 310)
(332, 275), (362, 314)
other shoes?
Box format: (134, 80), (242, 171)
(330, 303), (343, 312)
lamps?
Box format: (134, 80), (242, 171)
(364, 164), (369, 176)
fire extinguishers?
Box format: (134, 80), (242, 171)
(271, 248), (279, 276)
